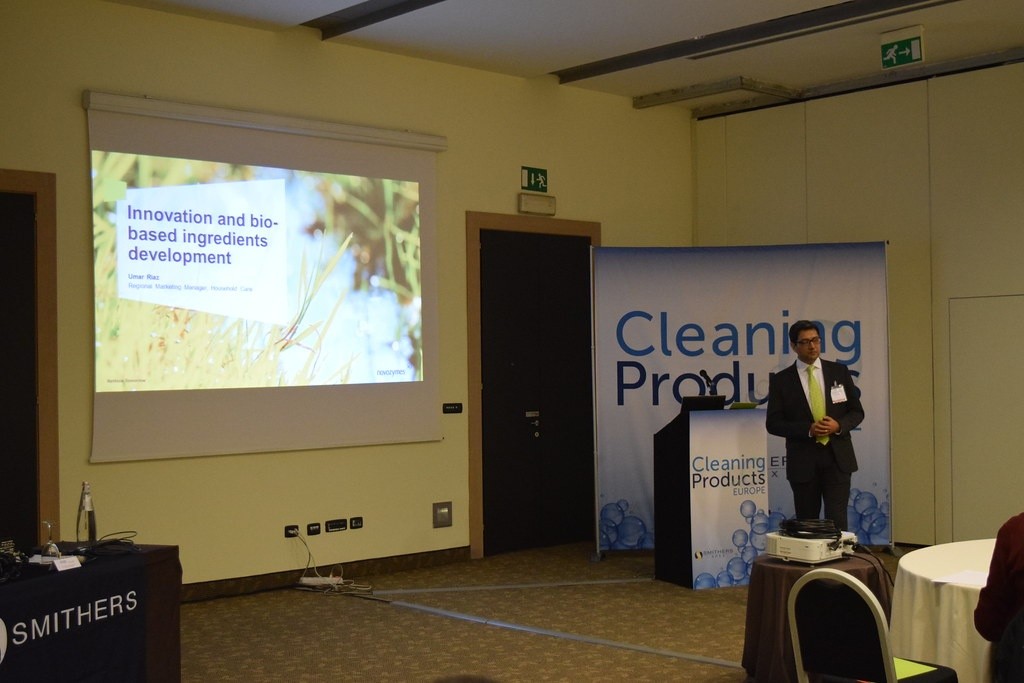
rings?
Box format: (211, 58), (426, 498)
(825, 430), (827, 432)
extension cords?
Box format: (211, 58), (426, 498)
(299, 577), (343, 585)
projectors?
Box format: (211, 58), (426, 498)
(766, 530), (857, 565)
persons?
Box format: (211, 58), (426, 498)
(972, 511), (1024, 643)
(765, 319), (865, 532)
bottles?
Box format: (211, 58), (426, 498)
(76, 480), (97, 543)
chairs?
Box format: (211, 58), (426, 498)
(787, 568), (960, 683)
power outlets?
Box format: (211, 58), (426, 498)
(284, 525), (299, 538)
(350, 517), (363, 528)
(307, 523), (320, 536)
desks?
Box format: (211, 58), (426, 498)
(893, 538), (1024, 683)
(742, 551), (890, 683)
(0, 539), (184, 683)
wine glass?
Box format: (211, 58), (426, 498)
(39, 519), (61, 563)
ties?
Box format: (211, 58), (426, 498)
(807, 365), (828, 445)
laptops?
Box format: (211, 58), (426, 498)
(681, 395), (726, 413)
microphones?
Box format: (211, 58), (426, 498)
(700, 369), (714, 386)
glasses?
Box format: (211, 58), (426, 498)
(794, 338), (822, 345)
